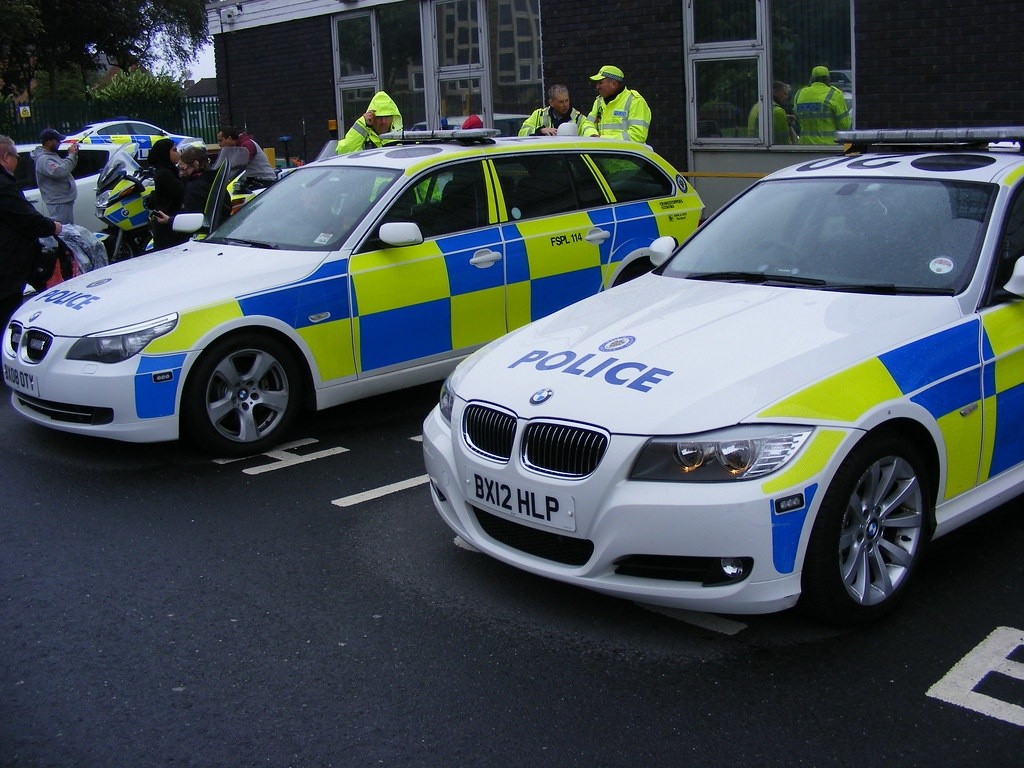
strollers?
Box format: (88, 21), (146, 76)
(36, 233), (110, 294)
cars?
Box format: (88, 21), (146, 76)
(416, 123), (1024, 633)
(13, 117), (340, 265)
(809, 69), (853, 120)
(0, 123), (707, 457)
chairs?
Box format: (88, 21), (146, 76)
(367, 183), (417, 241)
(549, 160), (617, 212)
(440, 164), (489, 228)
(516, 159), (565, 208)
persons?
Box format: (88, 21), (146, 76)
(147, 139), (233, 250)
(517, 84), (600, 137)
(30, 128), (80, 225)
(217, 126), (277, 187)
(794, 66), (851, 146)
(589, 65), (651, 142)
(335, 91), (403, 155)
(0, 135), (62, 350)
(748, 81), (793, 144)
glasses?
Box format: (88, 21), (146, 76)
(8, 152), (21, 161)
(181, 165), (192, 171)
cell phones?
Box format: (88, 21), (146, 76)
(149, 210), (162, 219)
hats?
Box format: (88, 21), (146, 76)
(812, 66), (829, 77)
(40, 129), (66, 140)
(590, 65), (624, 82)
